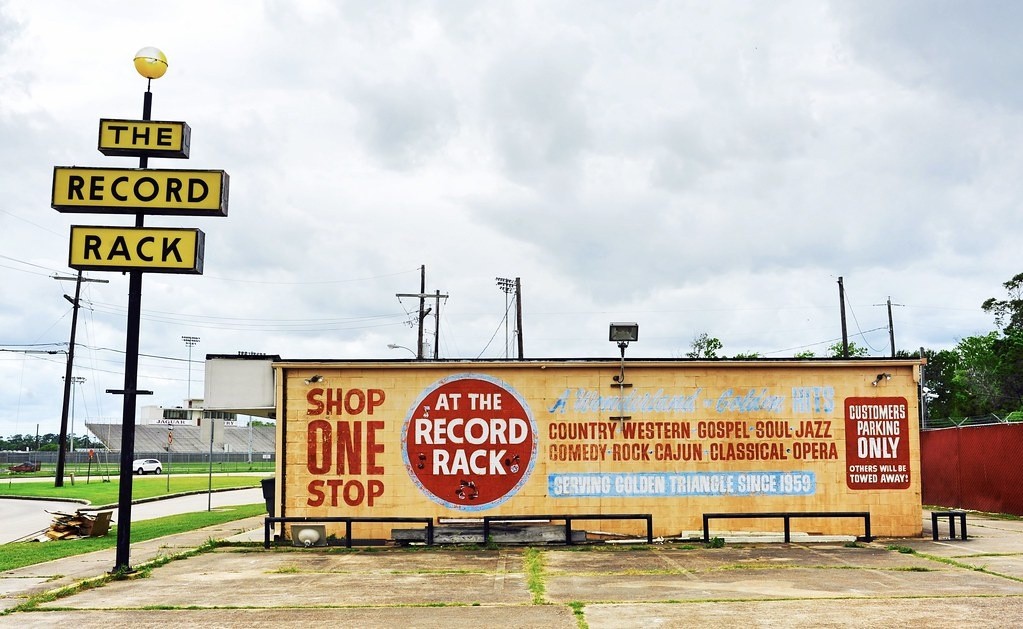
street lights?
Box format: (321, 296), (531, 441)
(495, 278), (517, 359)
(62, 375), (86, 453)
(182, 335), (201, 400)
(105, 45), (169, 574)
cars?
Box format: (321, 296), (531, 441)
(132, 459), (163, 476)
(8, 462), (36, 473)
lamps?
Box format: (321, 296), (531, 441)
(305, 375), (324, 386)
(609, 322), (638, 383)
(872, 373), (892, 387)
